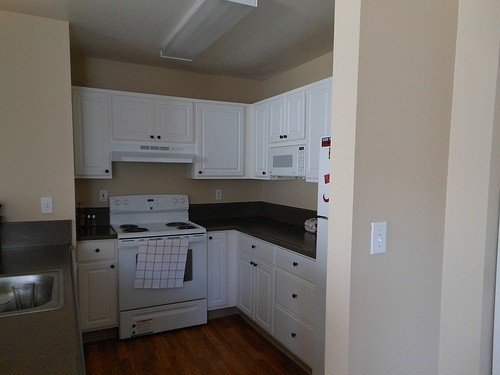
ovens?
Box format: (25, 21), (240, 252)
(116, 238), (208, 339)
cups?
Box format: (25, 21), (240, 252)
(12, 281), (38, 310)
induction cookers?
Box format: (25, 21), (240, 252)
(109, 195), (208, 239)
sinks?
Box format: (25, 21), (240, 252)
(0, 269), (65, 317)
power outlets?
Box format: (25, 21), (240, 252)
(40, 197), (52, 214)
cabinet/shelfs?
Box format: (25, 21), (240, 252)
(72, 76), (333, 183)
(76, 238), (119, 332)
(274, 246), (317, 369)
(236, 231), (276, 337)
(206, 231), (236, 310)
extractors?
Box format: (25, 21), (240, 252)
(110, 144), (195, 164)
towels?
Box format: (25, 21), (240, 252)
(133, 238), (189, 290)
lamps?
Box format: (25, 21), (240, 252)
(160, 0), (258, 62)
